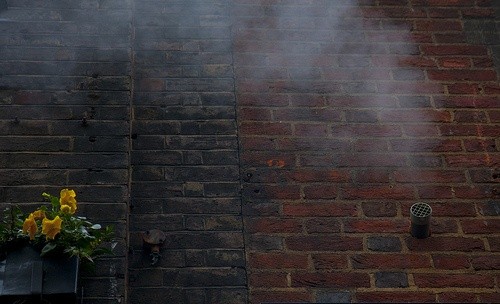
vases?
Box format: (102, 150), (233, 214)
(0, 238), (84, 303)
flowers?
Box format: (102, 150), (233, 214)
(1, 189), (119, 262)
